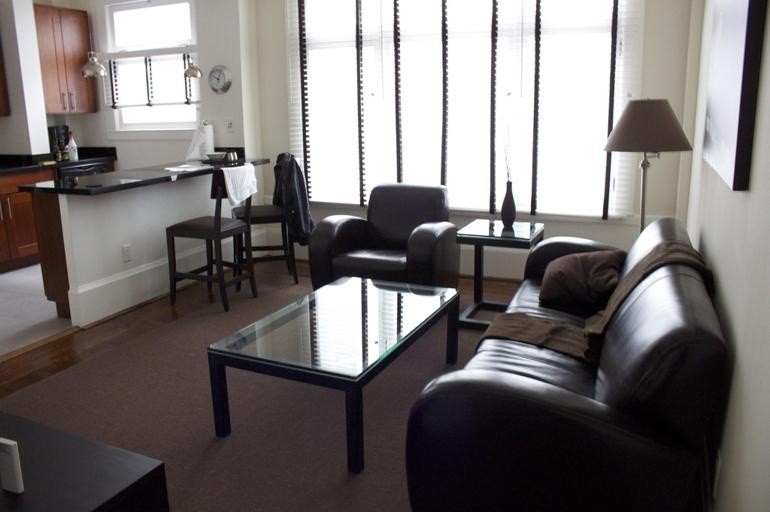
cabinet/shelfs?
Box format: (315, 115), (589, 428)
(0, 162), (58, 264)
(33, 3), (97, 117)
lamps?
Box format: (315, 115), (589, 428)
(602, 96), (694, 233)
(183, 51), (202, 81)
(79, 48), (108, 80)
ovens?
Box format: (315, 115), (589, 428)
(55, 157), (114, 180)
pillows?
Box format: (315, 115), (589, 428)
(539, 249), (629, 314)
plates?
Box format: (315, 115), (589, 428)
(201, 160), (224, 164)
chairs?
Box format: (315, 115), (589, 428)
(163, 162), (261, 311)
(56, 158), (105, 178)
(232, 152), (304, 290)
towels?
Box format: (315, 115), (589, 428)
(217, 163), (259, 206)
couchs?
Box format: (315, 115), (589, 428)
(306, 179), (462, 296)
(401, 213), (728, 512)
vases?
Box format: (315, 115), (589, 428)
(498, 179), (517, 229)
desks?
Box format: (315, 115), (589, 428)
(455, 216), (547, 331)
(0, 408), (172, 510)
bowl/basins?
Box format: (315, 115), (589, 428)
(205, 152), (225, 160)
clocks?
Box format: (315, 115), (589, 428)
(207, 64), (234, 96)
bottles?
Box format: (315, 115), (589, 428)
(67, 133), (78, 161)
(500, 181), (516, 227)
(62, 146), (69, 160)
(224, 151), (238, 164)
(55, 149), (62, 161)
